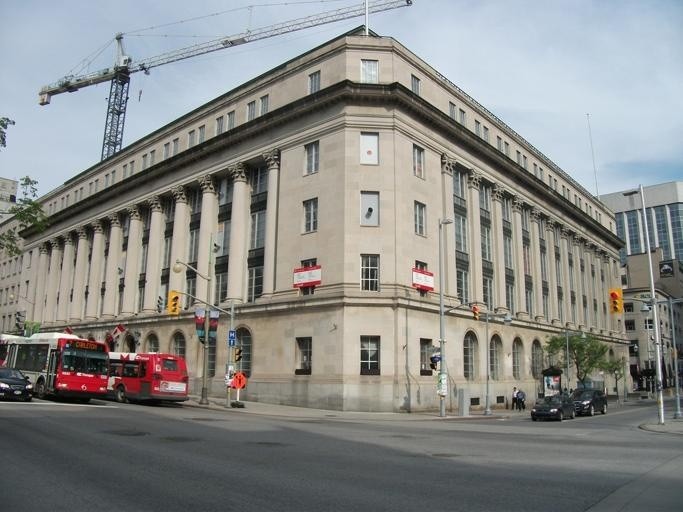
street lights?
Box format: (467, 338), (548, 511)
(437, 217), (453, 418)
(484, 314), (511, 415)
(621, 343), (638, 402)
(172, 231), (213, 405)
(639, 296), (681, 420)
(8, 292), (35, 324)
(622, 183), (664, 424)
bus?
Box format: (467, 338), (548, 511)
(90, 352), (188, 405)
(0, 332), (110, 403)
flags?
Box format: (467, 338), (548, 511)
(63, 326), (74, 335)
(208, 311), (219, 337)
(107, 324), (125, 342)
(193, 304), (206, 337)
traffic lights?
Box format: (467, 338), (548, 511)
(608, 287), (622, 314)
(171, 295), (178, 313)
(472, 306), (478, 320)
(234, 348), (242, 361)
(15, 311), (25, 329)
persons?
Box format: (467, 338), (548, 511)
(545, 376), (552, 389)
(569, 388), (573, 394)
(563, 387), (568, 394)
(516, 388), (526, 412)
(510, 386), (517, 410)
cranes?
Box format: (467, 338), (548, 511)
(37, 0), (414, 106)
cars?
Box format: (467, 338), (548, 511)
(530, 396), (576, 421)
(569, 388), (606, 416)
(0, 367), (33, 402)
